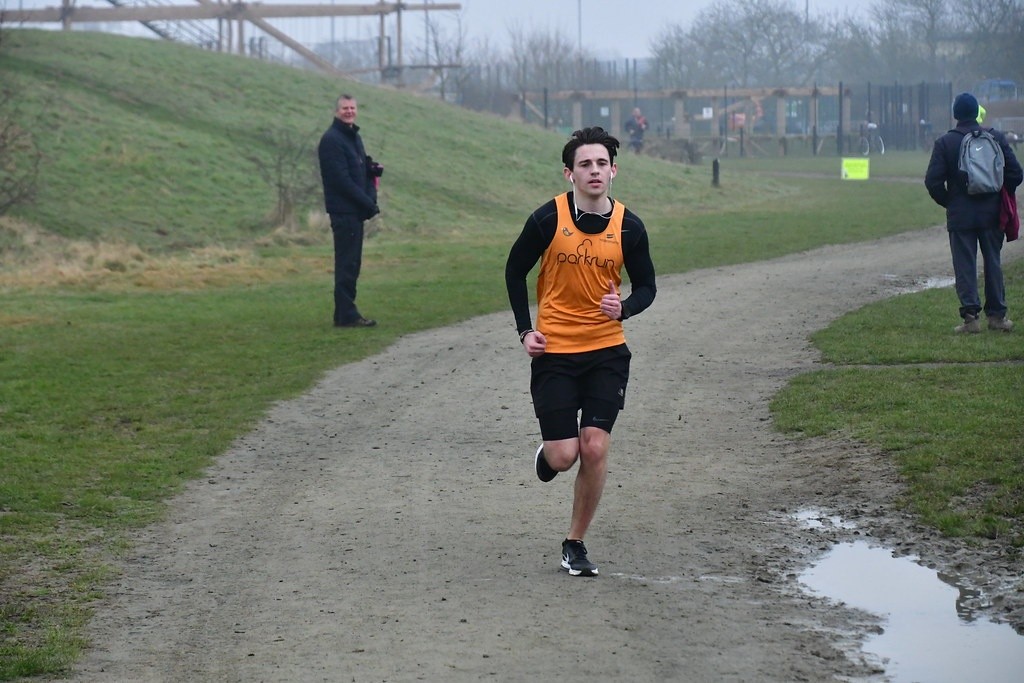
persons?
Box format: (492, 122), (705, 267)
(924, 93), (1023, 332)
(319, 94), (382, 328)
(506, 124), (656, 574)
(624, 107), (650, 156)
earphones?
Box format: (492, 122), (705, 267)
(569, 172), (575, 184)
(610, 170), (614, 184)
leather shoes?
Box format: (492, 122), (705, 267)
(333, 315), (378, 327)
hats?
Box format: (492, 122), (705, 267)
(953, 92), (979, 121)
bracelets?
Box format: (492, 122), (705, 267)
(520, 329), (533, 344)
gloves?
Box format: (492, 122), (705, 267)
(366, 202), (380, 219)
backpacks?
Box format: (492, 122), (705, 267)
(947, 127), (1006, 195)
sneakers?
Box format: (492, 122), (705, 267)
(533, 441), (560, 483)
(986, 316), (1016, 330)
(560, 538), (599, 576)
(953, 318), (982, 333)
